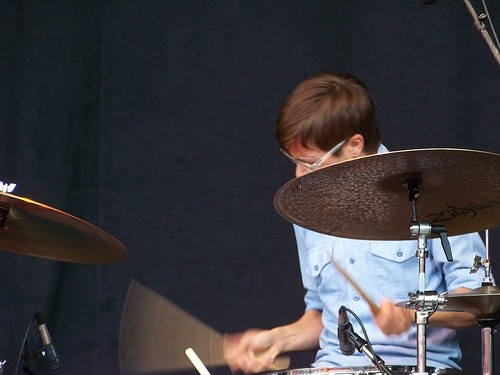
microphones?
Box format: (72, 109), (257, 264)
(337, 306), (355, 355)
(35, 312), (62, 369)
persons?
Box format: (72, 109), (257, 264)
(221, 72), (500, 374)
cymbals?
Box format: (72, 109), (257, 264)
(0, 191), (130, 269)
(396, 285), (500, 316)
(273, 149), (499, 242)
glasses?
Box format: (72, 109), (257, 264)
(279, 137), (348, 172)
